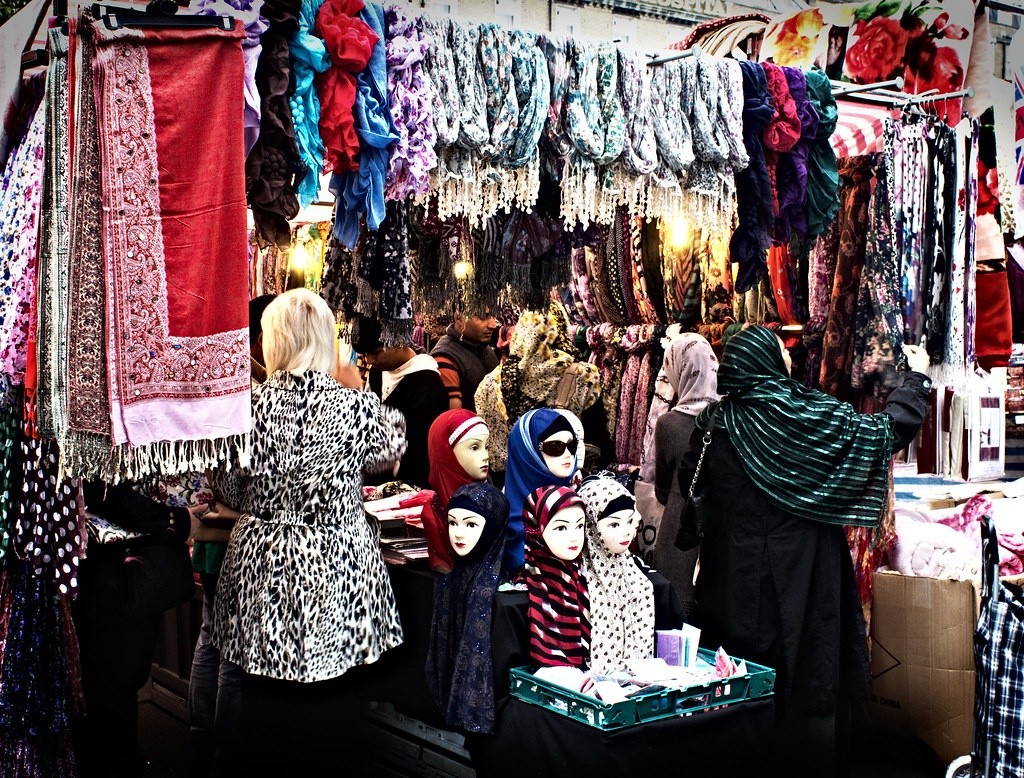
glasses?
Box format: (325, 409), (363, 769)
(538, 438), (579, 457)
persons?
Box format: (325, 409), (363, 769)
(351, 316), (449, 490)
(73, 286), (407, 778)
(656, 333), (721, 625)
(428, 408), (490, 480)
(502, 408), (578, 566)
(431, 295), (498, 413)
(577, 480), (635, 554)
(448, 483), (511, 559)
(475, 298), (613, 491)
(555, 408), (585, 477)
(679, 325), (932, 778)
(524, 485), (586, 560)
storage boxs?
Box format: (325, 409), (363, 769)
(869, 571), (1024, 762)
(507, 647), (777, 734)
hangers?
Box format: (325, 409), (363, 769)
(901, 86), (971, 130)
